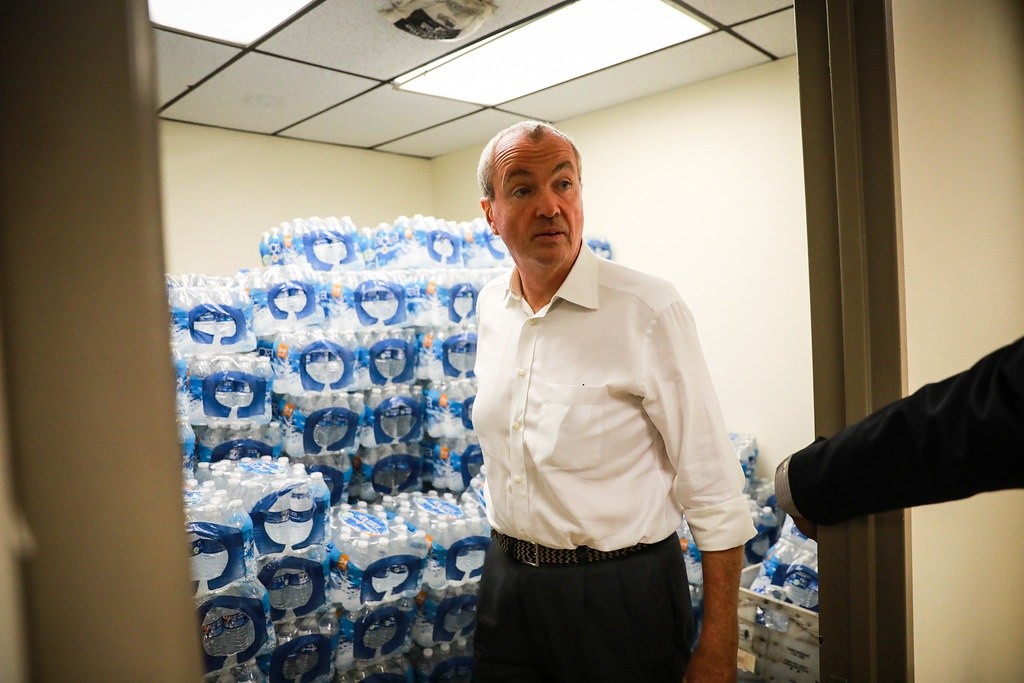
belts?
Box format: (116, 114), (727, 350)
(492, 529), (663, 568)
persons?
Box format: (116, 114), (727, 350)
(471, 120), (760, 683)
(775, 334), (1024, 541)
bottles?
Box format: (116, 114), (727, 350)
(162, 214), (818, 682)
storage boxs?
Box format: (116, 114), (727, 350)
(733, 563), (819, 683)
